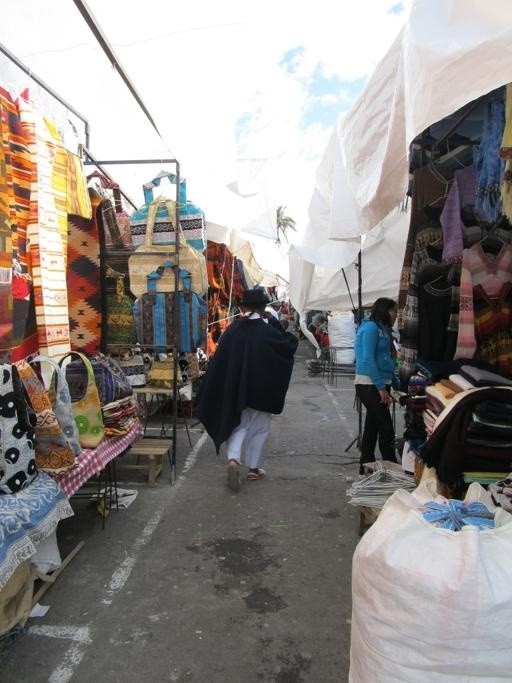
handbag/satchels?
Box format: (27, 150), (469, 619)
(34, 395), (105, 468)
(86, 169), (208, 390)
(65, 354), (133, 402)
(1, 364), (37, 494)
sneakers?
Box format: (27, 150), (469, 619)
(227, 460), (240, 490)
(248, 468), (265, 480)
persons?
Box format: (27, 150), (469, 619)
(353, 296), (399, 475)
(221, 287), (299, 491)
(277, 301), (290, 320)
(312, 313), (329, 346)
(262, 293), (290, 330)
